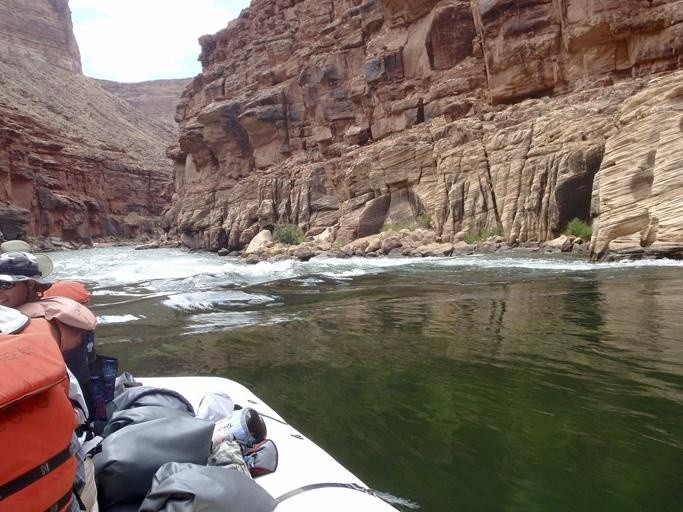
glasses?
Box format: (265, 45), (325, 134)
(1, 281), (26, 290)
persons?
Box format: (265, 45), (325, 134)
(0, 306), (93, 510)
(0, 239), (90, 309)
(0, 251), (98, 417)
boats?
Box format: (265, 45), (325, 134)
(83, 372), (406, 510)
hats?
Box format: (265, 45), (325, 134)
(0, 239), (54, 279)
(0, 251), (53, 292)
